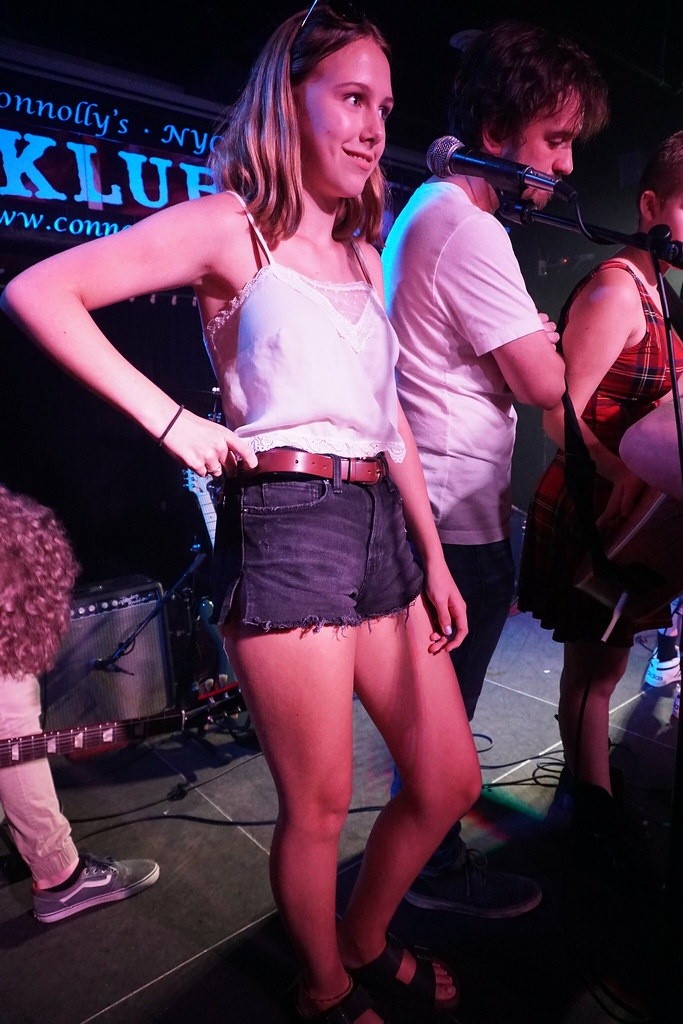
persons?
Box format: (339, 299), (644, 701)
(383, 18), (611, 920)
(516, 130), (683, 859)
(0, 485), (161, 923)
(0, 0), (482, 1024)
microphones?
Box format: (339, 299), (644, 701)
(424, 135), (577, 203)
(88, 657), (134, 676)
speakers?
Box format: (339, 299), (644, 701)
(40, 582), (179, 740)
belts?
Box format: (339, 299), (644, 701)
(236, 448), (387, 486)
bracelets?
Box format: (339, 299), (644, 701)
(159, 404), (184, 446)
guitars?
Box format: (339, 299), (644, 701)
(560, 483), (683, 624)
(0, 673), (247, 771)
(182, 466), (218, 550)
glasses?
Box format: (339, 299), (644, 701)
(300, 1), (382, 31)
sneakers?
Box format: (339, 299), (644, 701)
(31, 852), (161, 923)
(405, 849), (542, 919)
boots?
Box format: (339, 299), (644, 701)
(573, 779), (664, 904)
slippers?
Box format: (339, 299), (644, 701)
(290, 973), (390, 1024)
(345, 931), (460, 1009)
